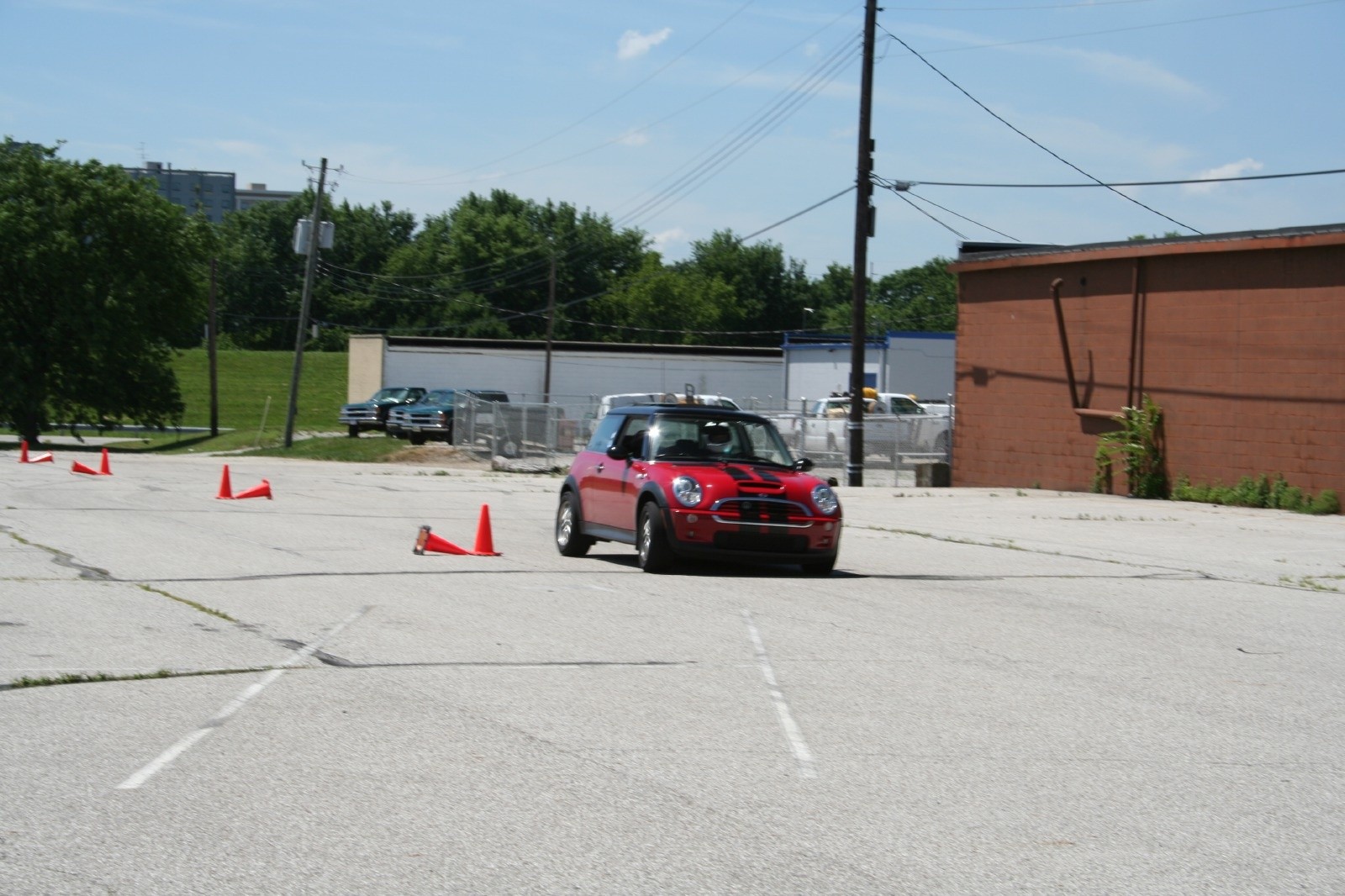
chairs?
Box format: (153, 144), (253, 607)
(670, 439), (701, 458)
(628, 430), (659, 457)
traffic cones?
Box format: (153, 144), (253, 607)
(31, 449), (54, 462)
(235, 479), (272, 499)
(214, 464), (234, 500)
(471, 503), (504, 556)
(100, 447), (113, 475)
(412, 525), (469, 556)
(71, 459), (98, 476)
(17, 440), (31, 463)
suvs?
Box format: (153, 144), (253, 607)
(337, 387), (426, 438)
(386, 388), (510, 445)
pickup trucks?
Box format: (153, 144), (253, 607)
(594, 386), (955, 468)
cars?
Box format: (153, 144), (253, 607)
(556, 402), (842, 576)
